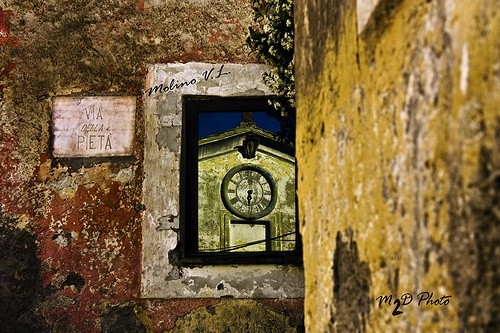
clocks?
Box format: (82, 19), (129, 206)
(221, 164), (279, 219)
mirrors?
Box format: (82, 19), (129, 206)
(181, 95), (303, 266)
